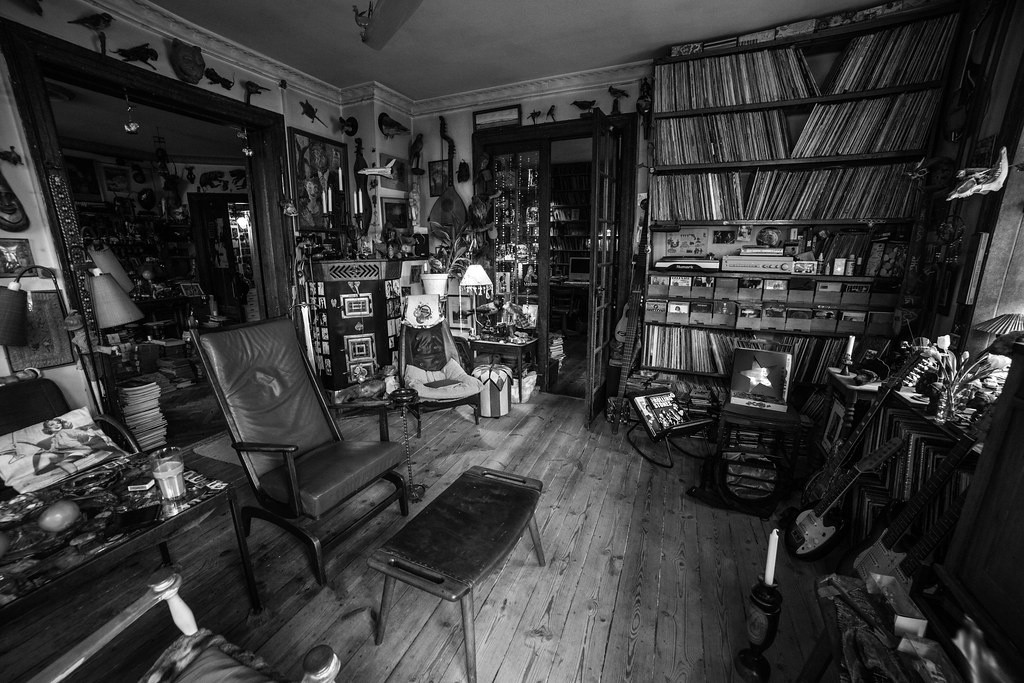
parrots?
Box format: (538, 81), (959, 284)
(465, 297), (527, 324)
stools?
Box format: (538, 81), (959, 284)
(471, 364), (514, 418)
(142, 321), (181, 341)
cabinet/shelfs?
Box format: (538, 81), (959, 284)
(523, 4), (1023, 548)
(78, 208), (208, 319)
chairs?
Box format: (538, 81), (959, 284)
(398, 294), (484, 438)
(187, 311), (407, 607)
(24, 571), (342, 683)
(0, 368), (141, 501)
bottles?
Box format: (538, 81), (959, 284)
(816, 252), (831, 275)
(209, 295), (217, 317)
(854, 257), (863, 275)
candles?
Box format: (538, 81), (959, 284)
(766, 529), (780, 584)
(846, 334), (855, 359)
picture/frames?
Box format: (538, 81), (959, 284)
(287, 127), (351, 233)
(0, 239), (38, 277)
(179, 283), (205, 297)
(427, 158), (451, 198)
(3, 289), (77, 374)
(381, 197), (411, 234)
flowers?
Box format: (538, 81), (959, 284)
(920, 335), (1012, 405)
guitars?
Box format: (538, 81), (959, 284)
(802, 352), (921, 519)
(614, 302), (630, 342)
(785, 437), (903, 563)
(835, 395), (1000, 596)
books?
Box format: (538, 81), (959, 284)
(117, 356), (195, 451)
(549, 175), (591, 275)
(154, 338), (185, 346)
(819, 233), (871, 276)
(641, 324), (849, 450)
(651, 15), (958, 221)
(851, 405), (977, 540)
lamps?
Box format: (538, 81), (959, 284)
(353, 0), (424, 50)
(242, 128), (254, 157)
(0, 265), (101, 412)
(121, 89), (141, 136)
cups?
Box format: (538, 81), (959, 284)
(148, 447), (186, 501)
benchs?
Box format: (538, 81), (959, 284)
(368, 465), (545, 683)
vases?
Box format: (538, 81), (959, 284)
(935, 391), (952, 424)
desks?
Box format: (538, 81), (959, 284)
(533, 277), (590, 324)
(472, 336), (538, 403)
(1, 444), (261, 619)
(328, 397), (421, 438)
